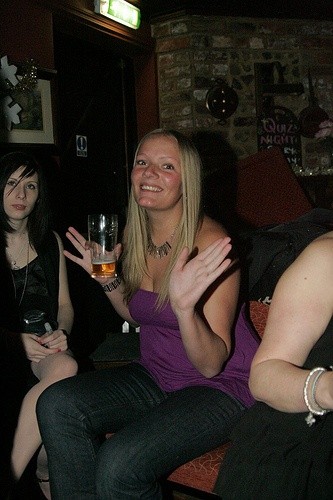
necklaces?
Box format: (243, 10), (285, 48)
(141, 206), (185, 257)
(5, 235), (30, 307)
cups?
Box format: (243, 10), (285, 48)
(87, 213), (119, 277)
(22, 310), (53, 338)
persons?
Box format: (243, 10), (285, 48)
(0, 150), (78, 500)
(36, 129), (261, 500)
(212, 232), (333, 500)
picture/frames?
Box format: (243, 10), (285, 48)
(0, 71), (63, 151)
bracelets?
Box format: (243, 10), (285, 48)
(4, 331), (11, 353)
(303, 365), (333, 427)
(101, 275), (123, 294)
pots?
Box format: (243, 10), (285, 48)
(296, 66), (329, 138)
(205, 79), (238, 126)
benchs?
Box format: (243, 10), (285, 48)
(106, 301), (269, 496)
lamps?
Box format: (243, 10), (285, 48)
(254, 61), (304, 119)
